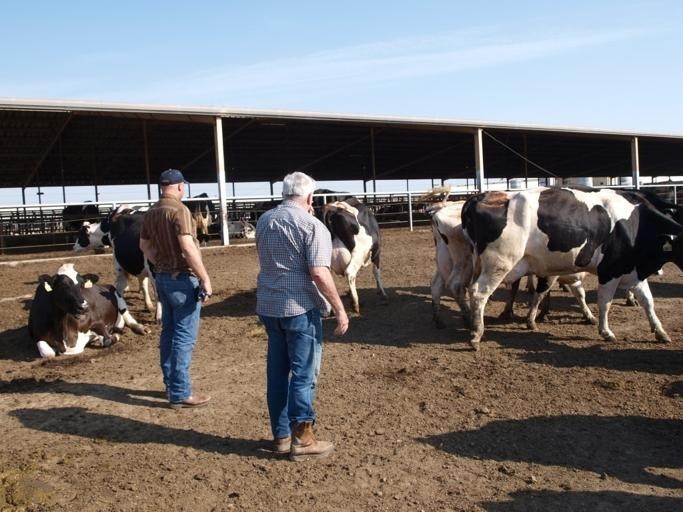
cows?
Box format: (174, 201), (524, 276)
(62, 197), (162, 322)
(318, 194), (389, 316)
(27, 263), (153, 359)
(413, 183), (683, 352)
(188, 192), (281, 248)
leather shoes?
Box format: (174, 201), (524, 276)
(170, 393), (211, 409)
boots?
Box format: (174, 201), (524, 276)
(274, 433), (293, 453)
(289, 420), (333, 461)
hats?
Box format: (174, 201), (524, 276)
(159, 169), (188, 186)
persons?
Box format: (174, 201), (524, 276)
(140, 169), (214, 410)
(256, 172), (350, 462)
